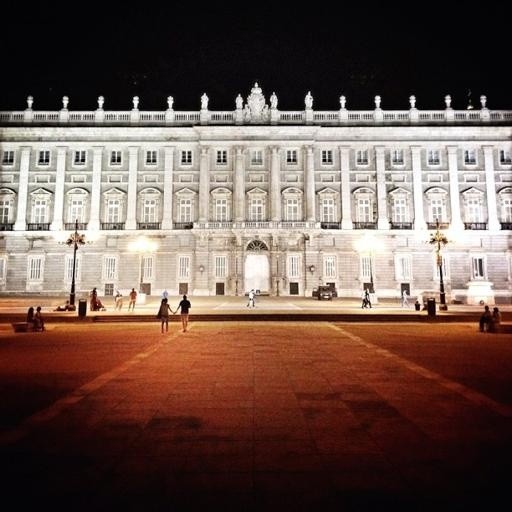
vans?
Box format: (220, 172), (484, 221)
(312, 286), (338, 302)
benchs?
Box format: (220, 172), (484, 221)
(486, 323), (512, 332)
(11, 322), (35, 332)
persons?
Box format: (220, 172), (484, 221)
(364, 288), (372, 308)
(173, 295), (192, 332)
(155, 297), (175, 334)
(401, 288), (409, 307)
(115, 290), (123, 311)
(487, 307), (502, 332)
(27, 287), (105, 332)
(362, 289), (369, 309)
(246, 289), (257, 308)
(127, 288), (137, 311)
(478, 305), (492, 334)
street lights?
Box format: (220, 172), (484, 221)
(427, 218), (448, 311)
(63, 219), (88, 311)
